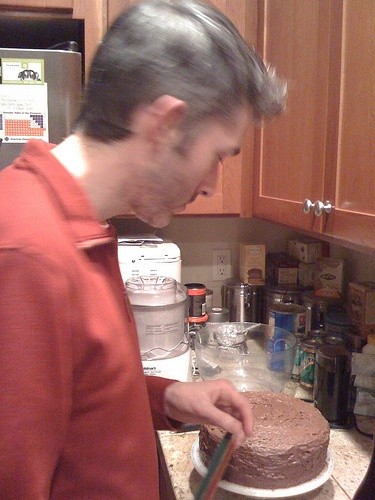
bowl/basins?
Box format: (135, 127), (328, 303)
(195, 322), (297, 391)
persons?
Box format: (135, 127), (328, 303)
(0, 0), (287, 500)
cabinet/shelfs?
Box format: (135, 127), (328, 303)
(71, 0), (375, 258)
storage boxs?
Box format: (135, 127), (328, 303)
(236, 235), (349, 300)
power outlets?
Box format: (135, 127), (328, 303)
(212, 248), (234, 282)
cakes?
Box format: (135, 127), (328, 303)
(199, 390), (329, 490)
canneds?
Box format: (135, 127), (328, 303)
(266, 302), (307, 375)
(281, 313), (353, 426)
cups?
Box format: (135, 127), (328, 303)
(185, 283), (206, 316)
(224, 283), (256, 323)
(184, 316), (208, 348)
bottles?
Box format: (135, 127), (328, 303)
(356, 334), (375, 436)
(315, 348), (350, 427)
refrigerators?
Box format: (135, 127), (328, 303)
(0, 49), (83, 173)
